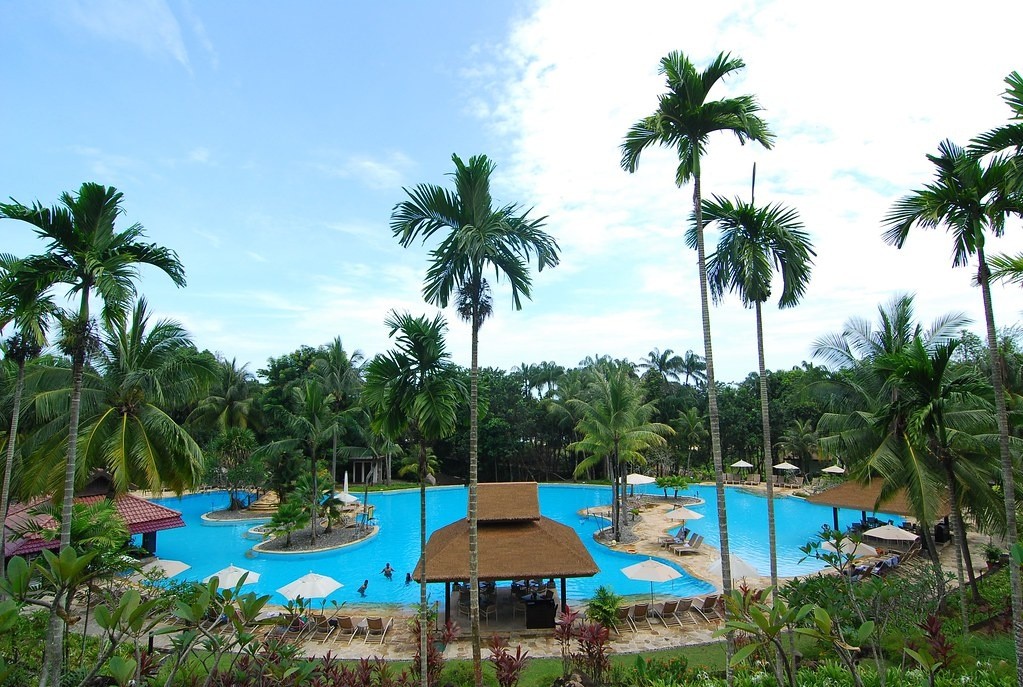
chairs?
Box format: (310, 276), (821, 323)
(453, 582), (554, 625)
(608, 588), (764, 636)
(657, 526), (704, 557)
(840, 514), (918, 581)
(724, 478), (803, 489)
(78, 580), (394, 645)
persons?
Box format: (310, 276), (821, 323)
(406, 573), (411, 581)
(547, 578), (556, 588)
(382, 563), (394, 573)
(362, 580), (368, 588)
(452, 582), (460, 591)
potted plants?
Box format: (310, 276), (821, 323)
(978, 542), (1002, 571)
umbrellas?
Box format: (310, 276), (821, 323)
(335, 492), (357, 502)
(276, 571), (345, 630)
(773, 461), (799, 469)
(863, 525), (919, 540)
(822, 465), (844, 473)
(615, 473), (656, 485)
(666, 506), (704, 531)
(822, 537), (877, 556)
(621, 557), (683, 618)
(140, 557), (192, 579)
(731, 459), (753, 468)
(204, 563), (261, 590)
(344, 471), (348, 494)
